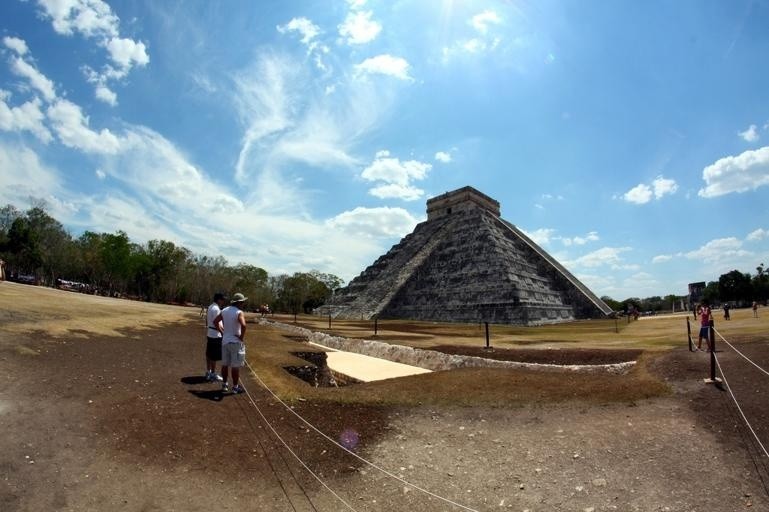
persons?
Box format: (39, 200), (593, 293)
(214, 292), (250, 395)
(695, 301), (713, 354)
(205, 292), (225, 383)
(752, 301), (760, 318)
(723, 303), (731, 321)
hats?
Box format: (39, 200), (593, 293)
(213, 293), (228, 300)
(230, 293), (248, 304)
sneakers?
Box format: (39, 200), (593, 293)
(231, 385), (245, 394)
(205, 371), (224, 381)
(222, 384), (229, 391)
